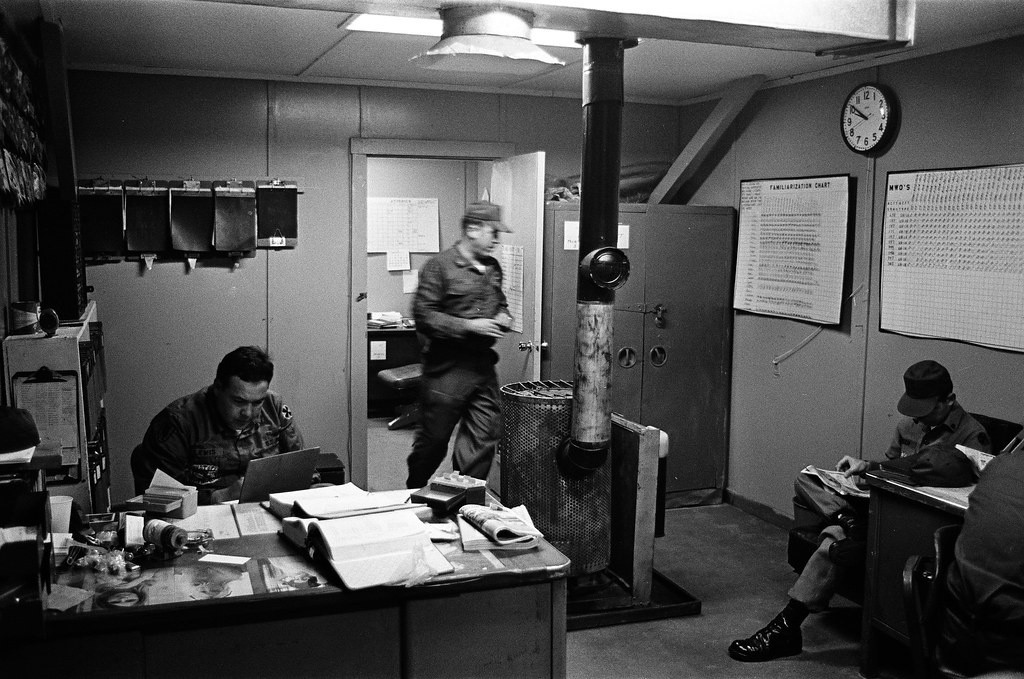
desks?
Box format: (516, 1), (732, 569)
(366, 318), (416, 414)
(859, 470), (977, 679)
(39, 482), (570, 679)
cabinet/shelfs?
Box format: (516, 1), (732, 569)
(4, 298), (111, 516)
(544, 204), (734, 509)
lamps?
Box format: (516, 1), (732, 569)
(405, 3), (564, 74)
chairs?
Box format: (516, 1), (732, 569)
(788, 410), (1024, 605)
(901, 553), (1024, 679)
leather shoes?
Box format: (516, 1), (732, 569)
(728, 613), (803, 662)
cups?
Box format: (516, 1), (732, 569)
(10, 301), (41, 334)
(49, 495), (74, 534)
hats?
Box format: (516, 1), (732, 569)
(464, 199), (510, 232)
(897, 359), (953, 418)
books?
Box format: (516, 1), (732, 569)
(457, 502), (543, 550)
(260, 481), (455, 591)
(367, 312), (416, 329)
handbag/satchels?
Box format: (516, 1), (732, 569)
(876, 441), (981, 487)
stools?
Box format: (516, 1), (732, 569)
(379, 363), (425, 430)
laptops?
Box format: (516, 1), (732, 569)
(219, 445), (323, 506)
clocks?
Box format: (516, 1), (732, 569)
(840, 82), (897, 155)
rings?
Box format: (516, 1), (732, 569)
(507, 317), (513, 320)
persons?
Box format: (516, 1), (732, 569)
(727, 360), (990, 662)
(946, 449), (1024, 679)
(406, 200), (513, 489)
(143, 346), (304, 504)
